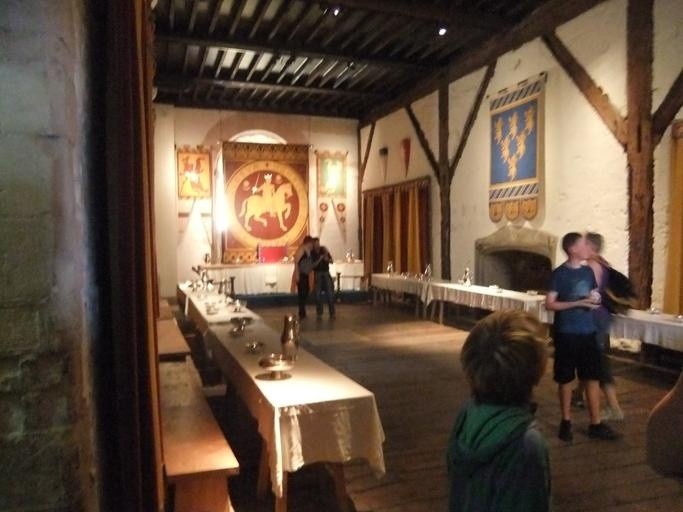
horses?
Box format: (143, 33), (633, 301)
(238, 181), (293, 232)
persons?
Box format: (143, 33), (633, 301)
(289, 236), (321, 320)
(570, 233), (626, 420)
(542, 234), (622, 442)
(647, 362), (683, 477)
(446, 308), (550, 511)
(309, 240), (337, 320)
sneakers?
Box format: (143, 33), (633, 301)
(600, 408), (623, 421)
(559, 419), (572, 441)
(587, 423), (623, 440)
(571, 390), (584, 407)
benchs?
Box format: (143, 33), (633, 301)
(156, 297), (241, 511)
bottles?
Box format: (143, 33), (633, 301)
(385, 262), (393, 279)
(280, 314), (299, 369)
(423, 263), (431, 283)
(461, 267), (471, 287)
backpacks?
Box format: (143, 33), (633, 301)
(593, 256), (638, 314)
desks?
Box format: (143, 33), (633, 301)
(535, 298), (682, 366)
(175, 279), (375, 512)
(373, 272), (438, 321)
(200, 259), (365, 299)
(427, 279), (547, 345)
